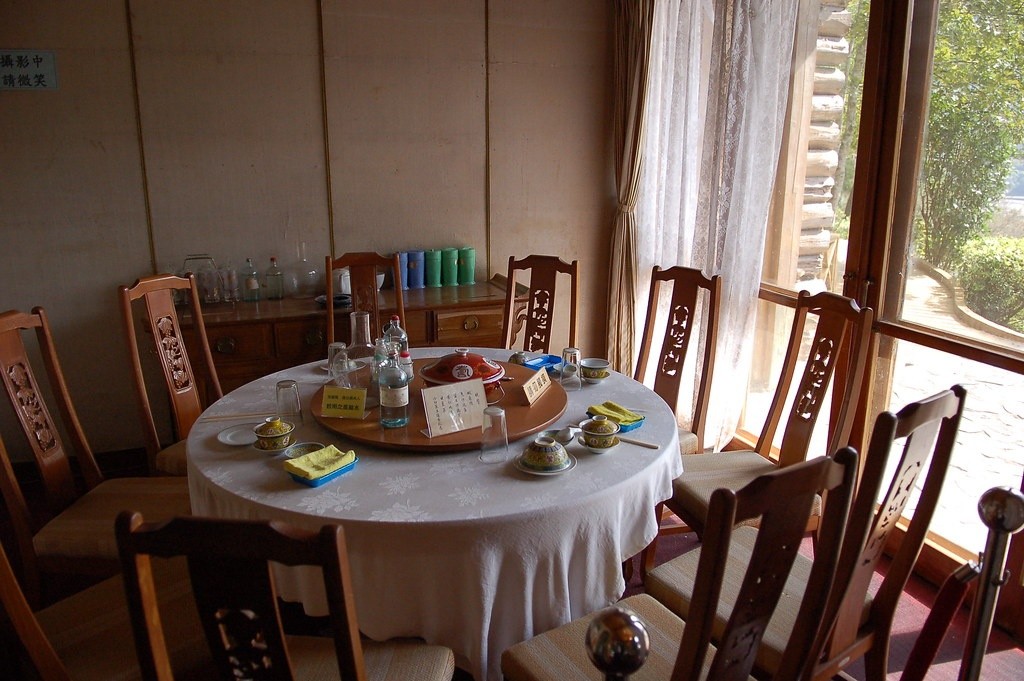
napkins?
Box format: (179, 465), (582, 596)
(587, 401), (644, 426)
(282, 444), (355, 480)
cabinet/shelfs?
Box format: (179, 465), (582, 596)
(179, 272), (526, 415)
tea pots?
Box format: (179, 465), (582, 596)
(333, 268), (351, 294)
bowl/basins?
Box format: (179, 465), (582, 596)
(580, 358), (610, 378)
(418, 348), (505, 405)
(508, 351), (531, 365)
(285, 441), (325, 459)
(518, 437), (571, 472)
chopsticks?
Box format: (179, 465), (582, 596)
(200, 411), (276, 422)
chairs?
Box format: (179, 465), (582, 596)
(1, 252), (1024, 681)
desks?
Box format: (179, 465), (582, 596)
(176, 343), (688, 681)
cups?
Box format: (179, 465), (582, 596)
(376, 272), (385, 293)
(327, 342), (347, 383)
(559, 347), (582, 391)
(392, 246), (476, 291)
(479, 406), (509, 463)
(276, 380), (304, 431)
(579, 415), (621, 448)
(253, 416), (295, 449)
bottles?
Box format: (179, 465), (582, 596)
(347, 311), (414, 428)
(221, 240), (320, 302)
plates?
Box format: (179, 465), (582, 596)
(512, 453), (577, 475)
(320, 360), (366, 371)
(217, 422), (262, 445)
(538, 430), (620, 453)
(255, 434), (298, 455)
(553, 363), (610, 383)
